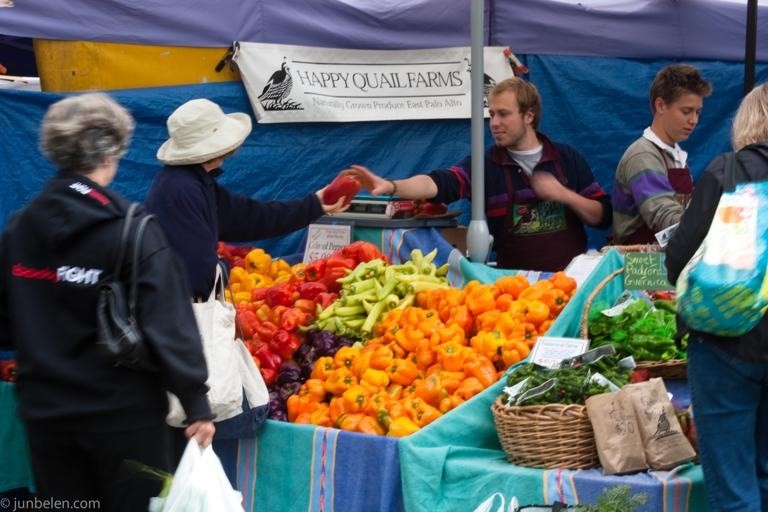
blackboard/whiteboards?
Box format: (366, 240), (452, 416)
(623, 252), (676, 291)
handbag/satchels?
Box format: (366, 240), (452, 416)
(96, 201), (157, 370)
(164, 262), (271, 441)
(675, 180), (768, 338)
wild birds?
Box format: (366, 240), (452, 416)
(258, 62), (292, 105)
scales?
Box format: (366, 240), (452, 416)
(329, 195), (422, 219)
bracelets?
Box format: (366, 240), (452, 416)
(385, 179), (397, 199)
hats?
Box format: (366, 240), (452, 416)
(156, 98), (252, 167)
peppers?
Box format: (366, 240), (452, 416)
(0, 360), (16, 382)
(219, 172), (689, 439)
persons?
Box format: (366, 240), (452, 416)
(1, 89), (221, 512)
(344, 73), (613, 274)
(143, 95), (353, 494)
(609, 61), (715, 248)
(659, 80), (768, 512)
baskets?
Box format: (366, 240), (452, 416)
(490, 394), (599, 470)
(580, 265), (687, 378)
